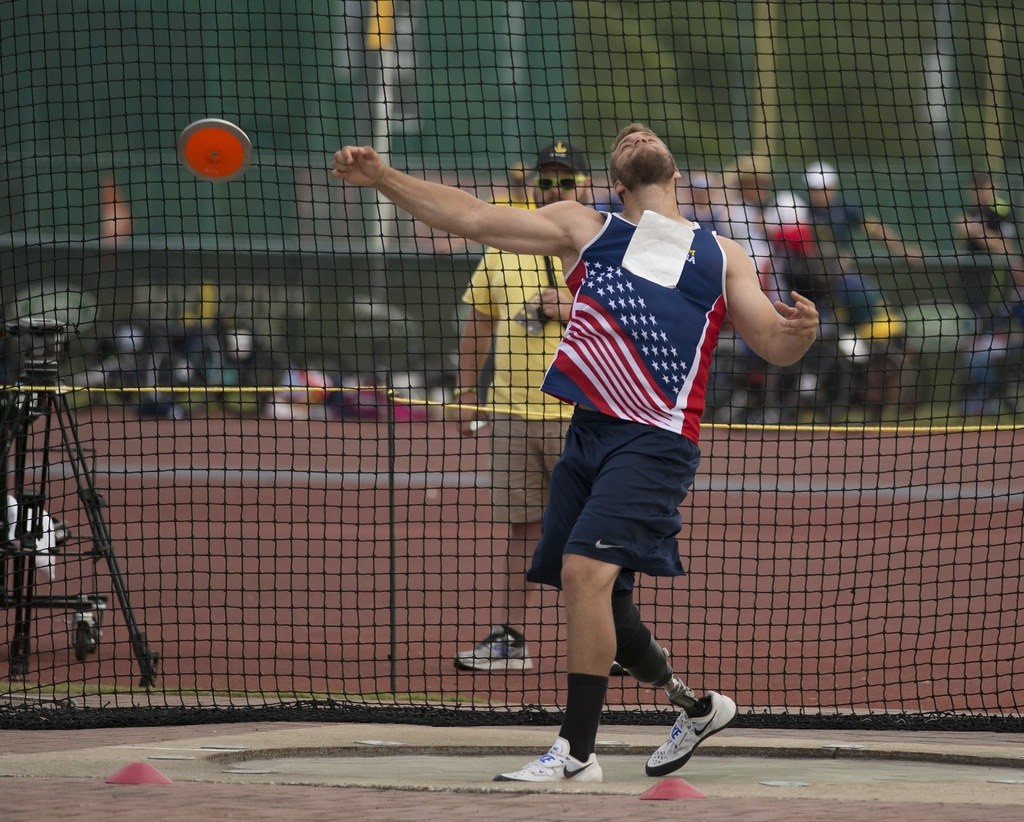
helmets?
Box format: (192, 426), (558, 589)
(734, 151), (771, 180)
(803, 160), (836, 187)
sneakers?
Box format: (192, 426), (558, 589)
(454, 625), (533, 670)
(645, 690), (738, 777)
(493, 735), (603, 783)
(608, 660), (631, 676)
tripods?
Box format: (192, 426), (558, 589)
(0, 317), (156, 692)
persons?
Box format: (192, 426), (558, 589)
(455, 142), (592, 670)
(956, 171), (1024, 416)
(332, 123), (819, 783)
(487, 161), (538, 209)
(686, 152), (924, 423)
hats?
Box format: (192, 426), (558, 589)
(536, 140), (589, 173)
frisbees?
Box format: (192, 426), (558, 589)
(180, 119), (252, 182)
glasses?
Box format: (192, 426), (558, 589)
(539, 178), (585, 191)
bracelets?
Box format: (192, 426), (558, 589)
(454, 386), (478, 393)
